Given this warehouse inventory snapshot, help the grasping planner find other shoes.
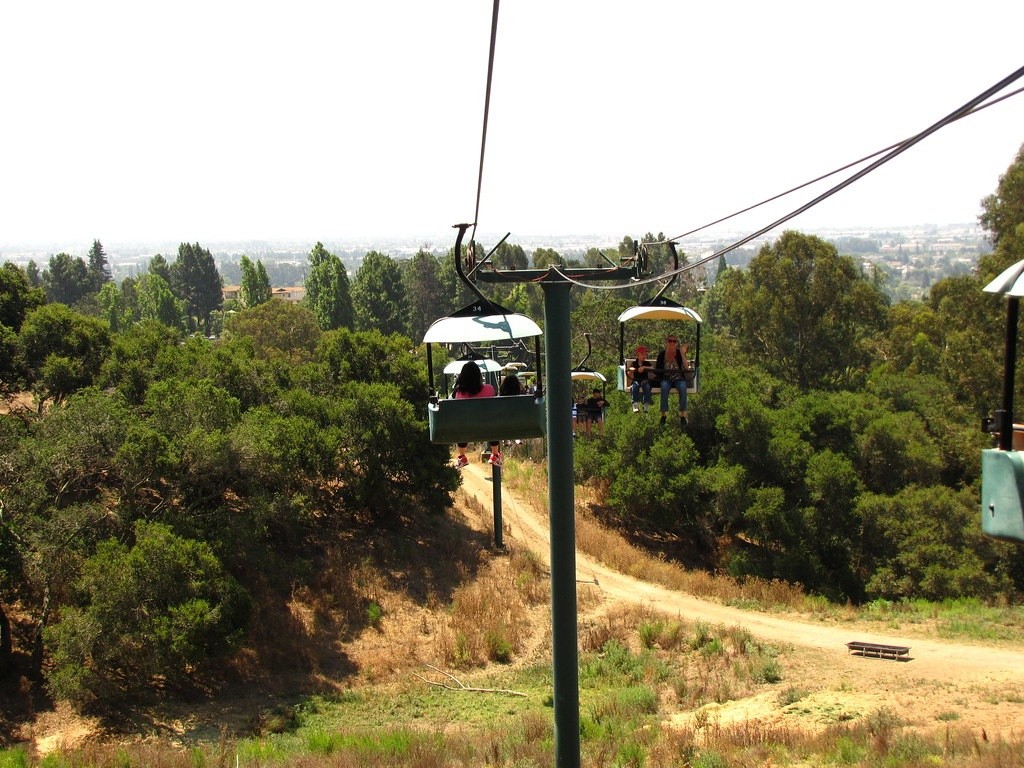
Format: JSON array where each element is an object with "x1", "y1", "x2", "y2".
[
  {"x1": 660, "y1": 415, "x2": 666, "y2": 425},
  {"x1": 633, "y1": 403, "x2": 639, "y2": 413},
  {"x1": 643, "y1": 403, "x2": 649, "y2": 412},
  {"x1": 680, "y1": 415, "x2": 688, "y2": 425}
]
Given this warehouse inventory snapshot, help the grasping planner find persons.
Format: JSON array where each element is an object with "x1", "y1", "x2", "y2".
[
  {"x1": 450, "y1": 362, "x2": 503, "y2": 468},
  {"x1": 629, "y1": 346, "x2": 654, "y2": 412},
  {"x1": 497, "y1": 375, "x2": 527, "y2": 446},
  {"x1": 654, "y1": 335, "x2": 690, "y2": 424},
  {"x1": 571, "y1": 389, "x2": 610, "y2": 438}
]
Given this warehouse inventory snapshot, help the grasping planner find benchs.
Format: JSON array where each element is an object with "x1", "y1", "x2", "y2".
[{"x1": 846, "y1": 641, "x2": 909, "y2": 660}]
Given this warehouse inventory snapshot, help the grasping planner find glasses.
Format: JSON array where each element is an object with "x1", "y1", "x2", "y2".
[{"x1": 667, "y1": 339, "x2": 677, "y2": 343}]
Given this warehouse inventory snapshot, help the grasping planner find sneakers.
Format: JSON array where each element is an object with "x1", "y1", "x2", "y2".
[
  {"x1": 453, "y1": 456, "x2": 469, "y2": 468},
  {"x1": 488, "y1": 453, "x2": 502, "y2": 466}
]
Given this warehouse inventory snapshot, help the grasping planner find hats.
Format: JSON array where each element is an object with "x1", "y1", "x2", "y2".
[
  {"x1": 636, "y1": 347, "x2": 649, "y2": 353},
  {"x1": 592, "y1": 388, "x2": 602, "y2": 393}
]
